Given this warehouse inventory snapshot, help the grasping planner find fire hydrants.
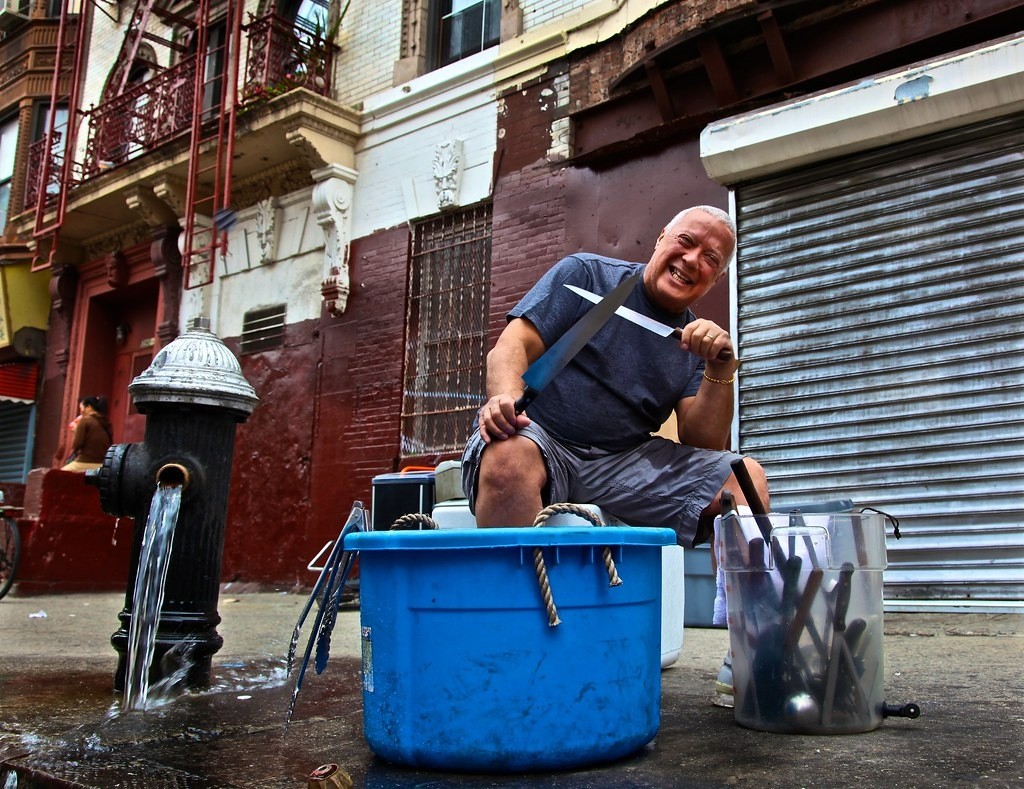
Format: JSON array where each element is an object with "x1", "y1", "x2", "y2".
[{"x1": 84, "y1": 320, "x2": 260, "y2": 696}]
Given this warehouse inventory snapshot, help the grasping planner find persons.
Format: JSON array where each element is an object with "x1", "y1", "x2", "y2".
[
  {"x1": 55, "y1": 395, "x2": 114, "y2": 473},
  {"x1": 463, "y1": 201, "x2": 818, "y2": 722}
]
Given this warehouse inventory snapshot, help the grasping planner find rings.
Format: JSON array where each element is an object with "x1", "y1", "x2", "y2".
[{"x1": 705, "y1": 334, "x2": 714, "y2": 340}]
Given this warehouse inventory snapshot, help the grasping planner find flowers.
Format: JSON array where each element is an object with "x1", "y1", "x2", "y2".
[{"x1": 235, "y1": 74, "x2": 300, "y2": 117}]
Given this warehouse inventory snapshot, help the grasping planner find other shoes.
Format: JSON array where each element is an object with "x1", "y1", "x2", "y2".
[{"x1": 710, "y1": 649, "x2": 734, "y2": 708}]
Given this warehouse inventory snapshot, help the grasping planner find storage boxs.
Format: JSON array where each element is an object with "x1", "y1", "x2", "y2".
[
  {"x1": 371, "y1": 474, "x2": 436, "y2": 530},
  {"x1": 683, "y1": 543, "x2": 729, "y2": 629},
  {"x1": 434, "y1": 460, "x2": 467, "y2": 502},
  {"x1": 433, "y1": 503, "x2": 684, "y2": 669}
]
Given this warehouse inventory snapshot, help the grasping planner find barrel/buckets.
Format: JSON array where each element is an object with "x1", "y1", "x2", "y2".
[{"x1": 718, "y1": 513, "x2": 888, "y2": 736}]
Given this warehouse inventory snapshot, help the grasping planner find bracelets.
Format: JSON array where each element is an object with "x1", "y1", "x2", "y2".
[{"x1": 702, "y1": 368, "x2": 736, "y2": 384}]
